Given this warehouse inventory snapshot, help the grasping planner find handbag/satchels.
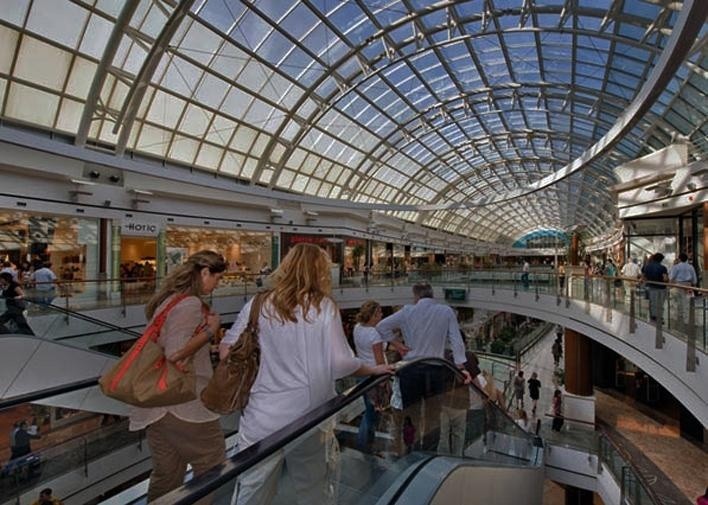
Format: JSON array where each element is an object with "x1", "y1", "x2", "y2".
[
  {"x1": 201, "y1": 332, "x2": 259, "y2": 415},
  {"x1": 375, "y1": 380, "x2": 393, "y2": 412},
  {"x1": 98, "y1": 326, "x2": 196, "y2": 407}
]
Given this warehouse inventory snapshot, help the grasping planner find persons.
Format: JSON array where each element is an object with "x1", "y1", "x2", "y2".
[
  {"x1": 1, "y1": 255, "x2": 157, "y2": 334},
  {"x1": 590, "y1": 251, "x2": 700, "y2": 325},
  {"x1": 224, "y1": 244, "x2": 395, "y2": 505},
  {"x1": 353, "y1": 280, "x2": 564, "y2": 458},
  {"x1": 7, "y1": 414, "x2": 122, "y2": 505},
  {"x1": 145, "y1": 250, "x2": 226, "y2": 505},
  {"x1": 256, "y1": 261, "x2": 270, "y2": 280},
  {"x1": 395, "y1": 257, "x2": 592, "y2": 299}
]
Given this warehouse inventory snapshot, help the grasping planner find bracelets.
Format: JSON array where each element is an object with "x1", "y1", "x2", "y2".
[{"x1": 206, "y1": 329, "x2": 215, "y2": 339}]
